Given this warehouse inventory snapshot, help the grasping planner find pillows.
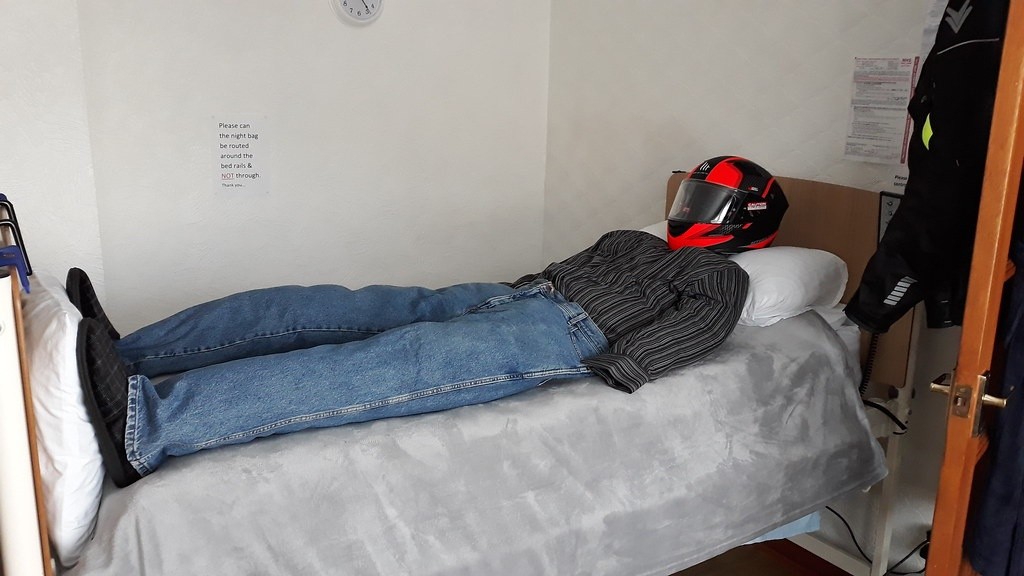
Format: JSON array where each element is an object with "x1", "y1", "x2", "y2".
[
  {"x1": 639, "y1": 219, "x2": 848, "y2": 327},
  {"x1": 21, "y1": 269, "x2": 107, "y2": 569}
]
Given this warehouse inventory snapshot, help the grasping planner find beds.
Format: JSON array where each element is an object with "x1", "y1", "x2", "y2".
[{"x1": 0, "y1": 171, "x2": 926, "y2": 576}]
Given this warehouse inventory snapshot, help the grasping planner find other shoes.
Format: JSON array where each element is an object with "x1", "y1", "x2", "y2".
[
  {"x1": 75, "y1": 320, "x2": 141, "y2": 487},
  {"x1": 66, "y1": 266, "x2": 119, "y2": 346}
]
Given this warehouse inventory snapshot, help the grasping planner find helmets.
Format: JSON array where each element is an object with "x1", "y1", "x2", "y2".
[{"x1": 666, "y1": 152, "x2": 790, "y2": 251}]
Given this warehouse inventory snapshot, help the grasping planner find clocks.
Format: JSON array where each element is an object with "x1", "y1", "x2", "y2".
[{"x1": 332, "y1": 0, "x2": 385, "y2": 27}]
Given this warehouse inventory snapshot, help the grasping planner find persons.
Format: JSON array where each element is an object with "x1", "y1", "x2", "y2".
[{"x1": 66, "y1": 155, "x2": 789, "y2": 487}]
